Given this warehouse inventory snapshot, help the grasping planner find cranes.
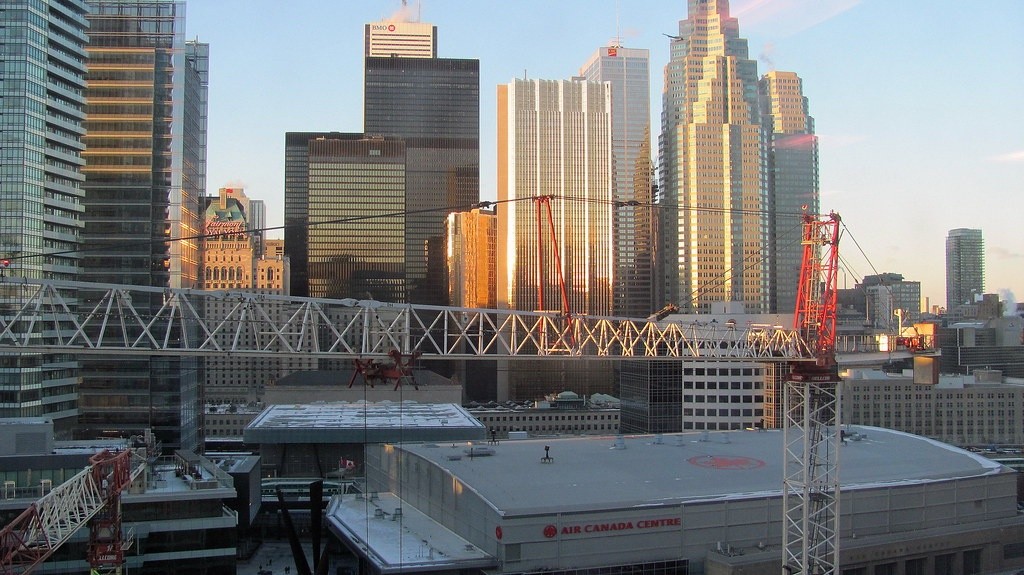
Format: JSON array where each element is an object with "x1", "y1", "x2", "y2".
[{"x1": 0, "y1": 192, "x2": 943, "y2": 574}]
